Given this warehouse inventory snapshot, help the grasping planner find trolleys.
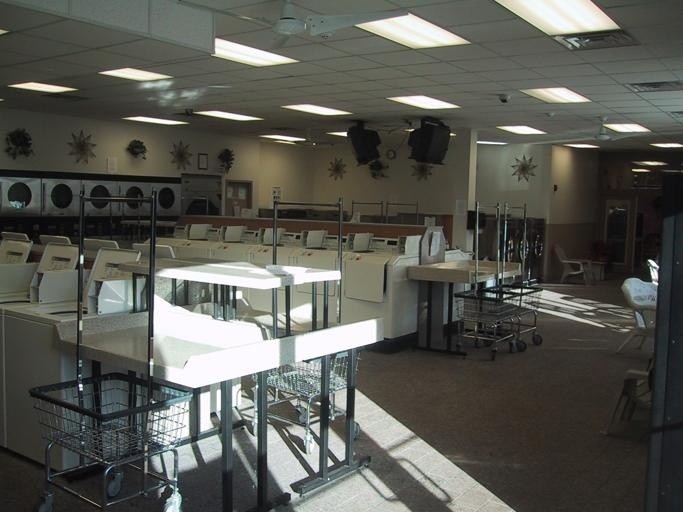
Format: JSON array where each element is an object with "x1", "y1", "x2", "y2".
[
  {"x1": 451, "y1": 284, "x2": 545, "y2": 363},
  {"x1": 28, "y1": 370, "x2": 193, "y2": 510},
  {"x1": 247, "y1": 347, "x2": 365, "y2": 457}
]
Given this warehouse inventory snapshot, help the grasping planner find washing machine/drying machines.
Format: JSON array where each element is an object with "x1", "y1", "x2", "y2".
[
  {"x1": 477, "y1": 215, "x2": 545, "y2": 284},
  {"x1": 0, "y1": 167, "x2": 475, "y2": 481}
]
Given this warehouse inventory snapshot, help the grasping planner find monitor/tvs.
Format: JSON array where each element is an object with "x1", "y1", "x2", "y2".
[
  {"x1": 343, "y1": 126, "x2": 382, "y2": 167},
  {"x1": 408, "y1": 125, "x2": 450, "y2": 165}
]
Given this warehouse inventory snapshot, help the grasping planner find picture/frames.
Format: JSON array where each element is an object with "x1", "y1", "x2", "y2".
[{"x1": 197, "y1": 153, "x2": 208, "y2": 170}]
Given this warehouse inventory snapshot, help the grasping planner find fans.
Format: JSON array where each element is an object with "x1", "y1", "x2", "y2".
[{"x1": 177, "y1": 0, "x2": 412, "y2": 53}]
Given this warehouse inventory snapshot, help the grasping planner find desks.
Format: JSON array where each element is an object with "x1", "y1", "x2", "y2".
[{"x1": 405, "y1": 258, "x2": 522, "y2": 357}]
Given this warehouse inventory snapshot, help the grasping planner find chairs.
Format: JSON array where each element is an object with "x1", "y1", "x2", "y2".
[
  {"x1": 551, "y1": 244, "x2": 593, "y2": 286},
  {"x1": 619, "y1": 276, "x2": 659, "y2": 351},
  {"x1": 646, "y1": 258, "x2": 660, "y2": 284}
]
